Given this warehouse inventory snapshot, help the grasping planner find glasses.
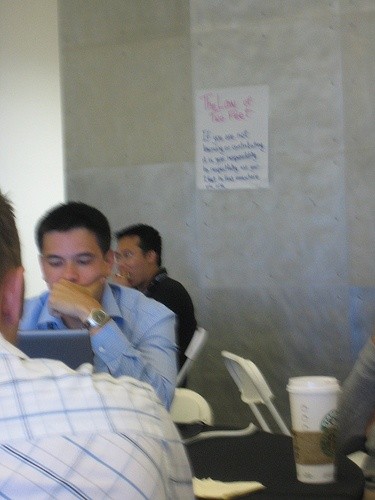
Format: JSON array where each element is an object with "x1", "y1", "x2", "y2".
[{"x1": 113, "y1": 247, "x2": 149, "y2": 265}]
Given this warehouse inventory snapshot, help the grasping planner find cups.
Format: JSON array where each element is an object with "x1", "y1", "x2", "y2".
[{"x1": 286, "y1": 374, "x2": 339, "y2": 485}]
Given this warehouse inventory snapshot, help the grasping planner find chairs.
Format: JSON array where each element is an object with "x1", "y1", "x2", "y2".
[{"x1": 168, "y1": 327, "x2": 291, "y2": 436}]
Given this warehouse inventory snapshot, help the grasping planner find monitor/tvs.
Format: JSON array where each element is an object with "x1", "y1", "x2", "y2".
[{"x1": 14, "y1": 329, "x2": 93, "y2": 370}]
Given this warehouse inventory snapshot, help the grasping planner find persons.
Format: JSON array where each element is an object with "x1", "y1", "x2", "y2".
[
  {"x1": 18, "y1": 201, "x2": 178, "y2": 411},
  {"x1": 322, "y1": 330, "x2": 375, "y2": 457},
  {"x1": 0, "y1": 194, "x2": 196, "y2": 500},
  {"x1": 113, "y1": 224, "x2": 197, "y2": 391}
]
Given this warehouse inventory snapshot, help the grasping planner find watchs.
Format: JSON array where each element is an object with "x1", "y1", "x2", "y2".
[{"x1": 84, "y1": 309, "x2": 110, "y2": 329}]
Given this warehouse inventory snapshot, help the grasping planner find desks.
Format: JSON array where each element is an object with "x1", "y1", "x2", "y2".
[{"x1": 177, "y1": 423, "x2": 365, "y2": 500}]
